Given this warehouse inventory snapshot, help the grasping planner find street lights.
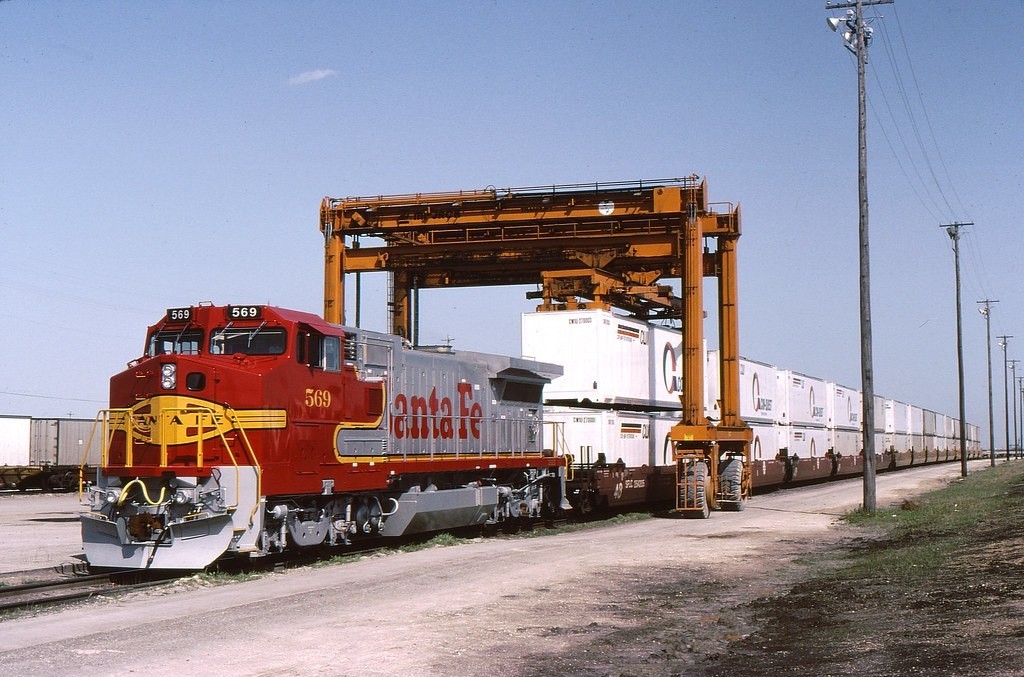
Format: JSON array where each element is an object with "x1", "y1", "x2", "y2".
[
  {"x1": 978, "y1": 309, "x2": 995, "y2": 468},
  {"x1": 998, "y1": 343, "x2": 1010, "y2": 462},
  {"x1": 1009, "y1": 364, "x2": 1021, "y2": 461},
  {"x1": 826, "y1": 14, "x2": 875, "y2": 518}
]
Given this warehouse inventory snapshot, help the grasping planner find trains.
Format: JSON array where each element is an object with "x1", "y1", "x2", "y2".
[{"x1": 56, "y1": 301, "x2": 980, "y2": 586}]
